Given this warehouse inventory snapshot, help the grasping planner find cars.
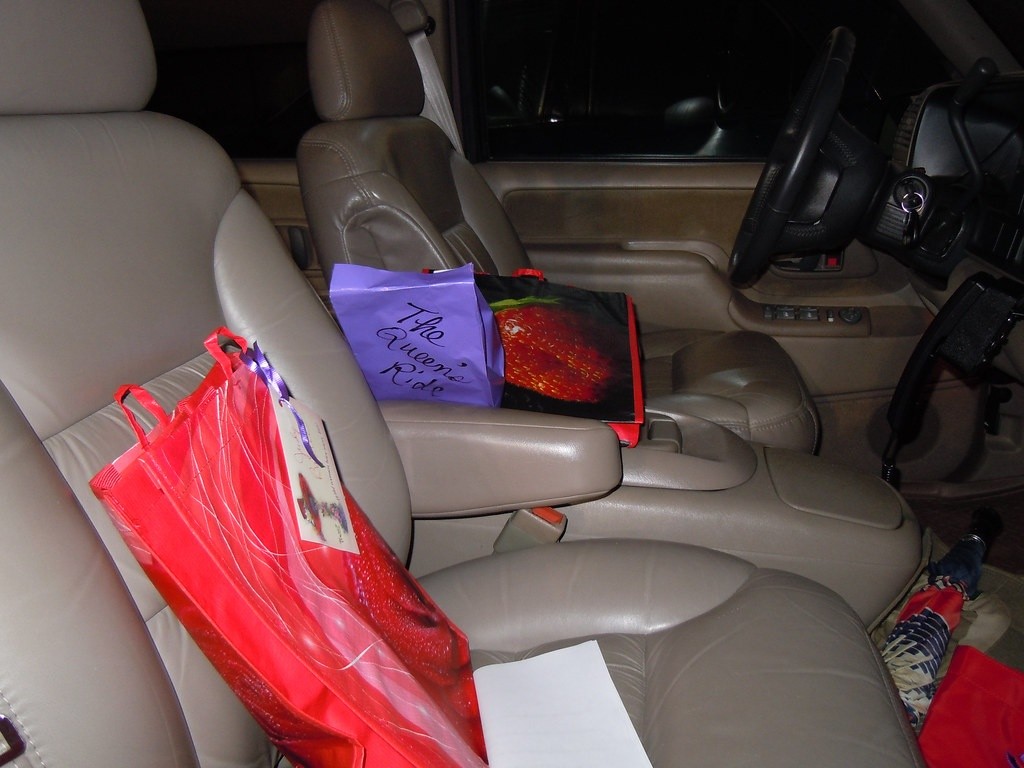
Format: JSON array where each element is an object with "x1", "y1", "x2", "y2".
[{"x1": 216, "y1": 0, "x2": 924, "y2": 155}]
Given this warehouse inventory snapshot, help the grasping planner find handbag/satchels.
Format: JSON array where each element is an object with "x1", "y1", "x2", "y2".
[
  {"x1": 421, "y1": 266, "x2": 644, "y2": 450},
  {"x1": 89, "y1": 324, "x2": 488, "y2": 768}
]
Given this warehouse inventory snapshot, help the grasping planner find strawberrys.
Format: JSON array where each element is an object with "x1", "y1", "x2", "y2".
[{"x1": 493, "y1": 304, "x2": 633, "y2": 404}]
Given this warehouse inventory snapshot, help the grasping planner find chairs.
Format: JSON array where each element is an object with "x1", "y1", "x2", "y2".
[{"x1": 0, "y1": 0, "x2": 927, "y2": 768}]
